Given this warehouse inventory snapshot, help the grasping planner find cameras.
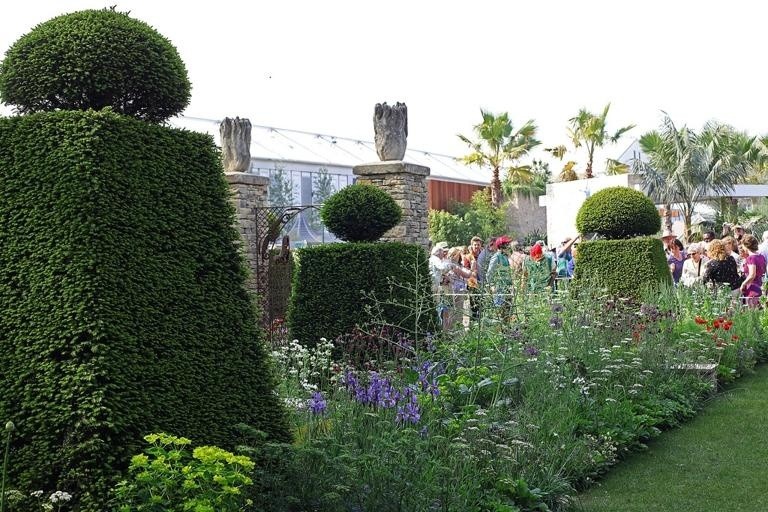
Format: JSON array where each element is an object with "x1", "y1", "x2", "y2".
[{"x1": 724, "y1": 223, "x2": 732, "y2": 228}]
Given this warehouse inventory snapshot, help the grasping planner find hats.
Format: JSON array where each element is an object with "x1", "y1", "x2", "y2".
[
  {"x1": 441, "y1": 242, "x2": 448, "y2": 252},
  {"x1": 496, "y1": 236, "x2": 513, "y2": 248},
  {"x1": 661, "y1": 230, "x2": 677, "y2": 238}
]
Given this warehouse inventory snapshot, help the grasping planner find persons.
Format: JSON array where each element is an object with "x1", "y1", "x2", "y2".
[
  {"x1": 662, "y1": 222, "x2": 768, "y2": 311},
  {"x1": 428, "y1": 234, "x2": 580, "y2": 334}
]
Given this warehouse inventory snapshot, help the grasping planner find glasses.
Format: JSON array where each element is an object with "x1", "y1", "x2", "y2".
[{"x1": 688, "y1": 253, "x2": 696, "y2": 256}]
